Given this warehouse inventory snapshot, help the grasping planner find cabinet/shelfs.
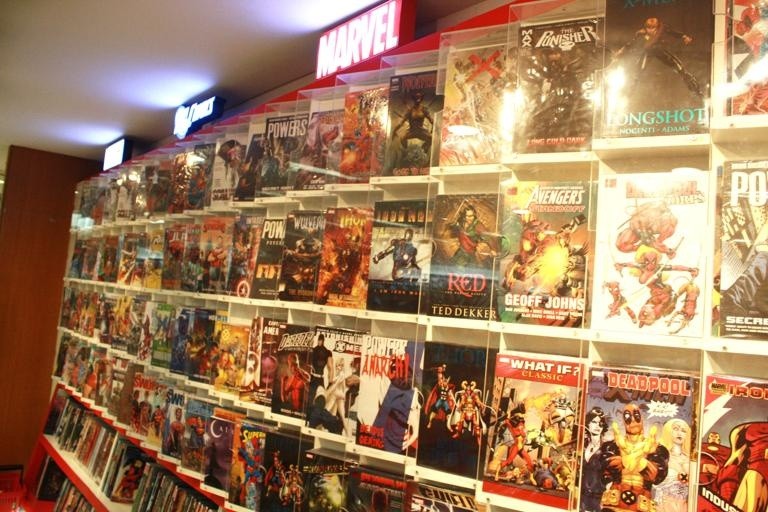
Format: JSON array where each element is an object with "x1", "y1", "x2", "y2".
[{"x1": 23, "y1": 0, "x2": 768, "y2": 511}]
